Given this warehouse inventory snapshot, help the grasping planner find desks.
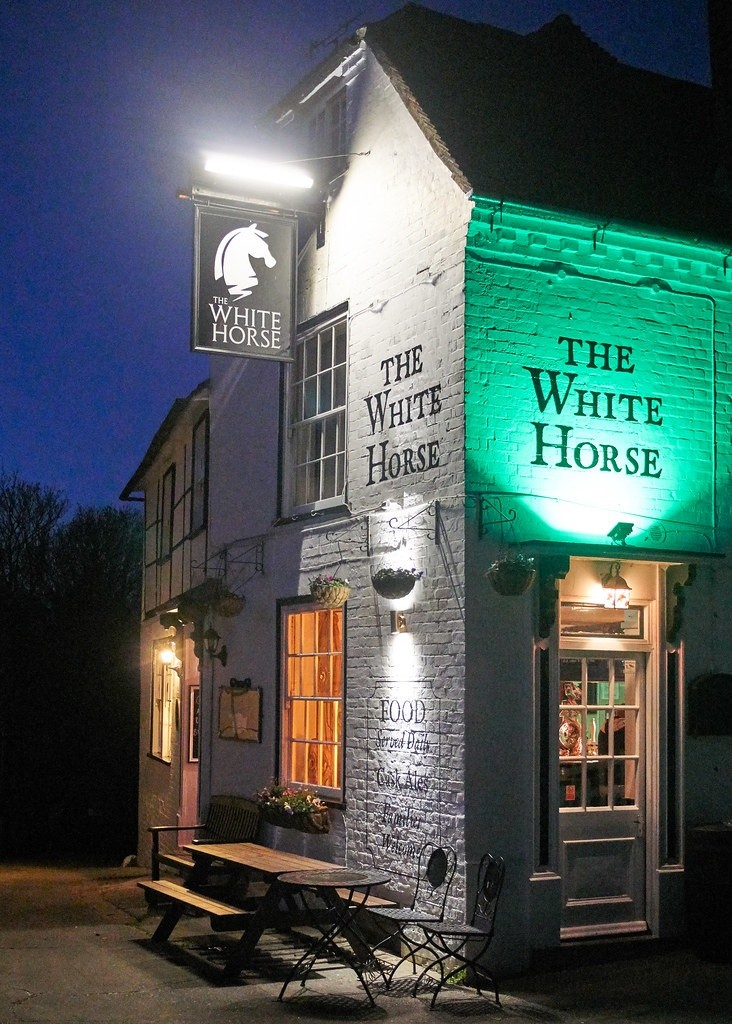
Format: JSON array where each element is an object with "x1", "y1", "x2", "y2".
[
  {"x1": 149, "y1": 842, "x2": 348, "y2": 982},
  {"x1": 276, "y1": 868, "x2": 391, "y2": 1008}
]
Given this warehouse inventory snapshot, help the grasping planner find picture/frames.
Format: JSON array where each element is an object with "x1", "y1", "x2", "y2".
[{"x1": 188, "y1": 684, "x2": 200, "y2": 763}]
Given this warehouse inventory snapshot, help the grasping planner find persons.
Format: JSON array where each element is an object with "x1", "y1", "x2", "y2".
[{"x1": 598, "y1": 703, "x2": 626, "y2": 806}]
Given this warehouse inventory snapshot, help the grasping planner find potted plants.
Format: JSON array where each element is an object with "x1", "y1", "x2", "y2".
[
  {"x1": 486, "y1": 553, "x2": 537, "y2": 597},
  {"x1": 177, "y1": 596, "x2": 208, "y2": 623},
  {"x1": 210, "y1": 586, "x2": 247, "y2": 617}
]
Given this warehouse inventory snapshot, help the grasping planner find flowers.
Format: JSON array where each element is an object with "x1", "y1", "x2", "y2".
[
  {"x1": 306, "y1": 573, "x2": 351, "y2": 588},
  {"x1": 254, "y1": 774, "x2": 328, "y2": 814},
  {"x1": 367, "y1": 567, "x2": 424, "y2": 580}
]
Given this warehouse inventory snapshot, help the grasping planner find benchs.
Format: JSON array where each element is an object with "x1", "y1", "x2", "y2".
[
  {"x1": 138, "y1": 879, "x2": 257, "y2": 982},
  {"x1": 310, "y1": 887, "x2": 398, "y2": 964}
]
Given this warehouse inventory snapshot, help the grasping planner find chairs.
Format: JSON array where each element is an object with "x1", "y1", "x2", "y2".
[
  {"x1": 412, "y1": 853, "x2": 506, "y2": 1010},
  {"x1": 144, "y1": 794, "x2": 261, "y2": 917},
  {"x1": 360, "y1": 841, "x2": 458, "y2": 993}
]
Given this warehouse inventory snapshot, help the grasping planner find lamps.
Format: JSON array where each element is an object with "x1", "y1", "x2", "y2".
[
  {"x1": 600, "y1": 561, "x2": 634, "y2": 612},
  {"x1": 203, "y1": 621, "x2": 228, "y2": 667},
  {"x1": 200, "y1": 150, "x2": 371, "y2": 190},
  {"x1": 389, "y1": 610, "x2": 406, "y2": 635},
  {"x1": 608, "y1": 520, "x2": 634, "y2": 547}
]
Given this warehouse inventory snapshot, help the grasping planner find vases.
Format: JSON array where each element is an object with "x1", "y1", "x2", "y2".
[
  {"x1": 372, "y1": 578, "x2": 416, "y2": 599},
  {"x1": 286, "y1": 812, "x2": 331, "y2": 835},
  {"x1": 258, "y1": 805, "x2": 292, "y2": 830},
  {"x1": 310, "y1": 588, "x2": 351, "y2": 608}
]
